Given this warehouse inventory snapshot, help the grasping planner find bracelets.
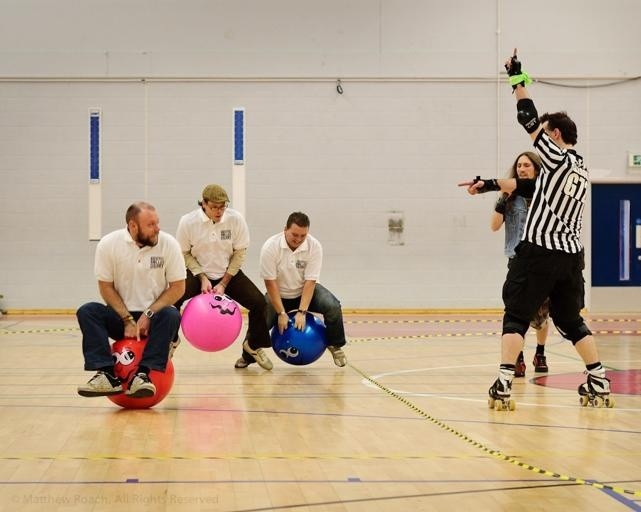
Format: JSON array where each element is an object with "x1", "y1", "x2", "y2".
[
  {"x1": 220, "y1": 281, "x2": 227, "y2": 288},
  {"x1": 121, "y1": 315, "x2": 135, "y2": 320},
  {"x1": 278, "y1": 311, "x2": 286, "y2": 315}
]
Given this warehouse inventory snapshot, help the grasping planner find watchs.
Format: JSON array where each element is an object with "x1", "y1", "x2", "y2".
[
  {"x1": 299, "y1": 309, "x2": 307, "y2": 315},
  {"x1": 144, "y1": 308, "x2": 155, "y2": 319}
]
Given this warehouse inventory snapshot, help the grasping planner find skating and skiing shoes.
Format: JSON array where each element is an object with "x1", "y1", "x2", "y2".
[
  {"x1": 577, "y1": 366, "x2": 615, "y2": 408},
  {"x1": 488, "y1": 369, "x2": 515, "y2": 411}
]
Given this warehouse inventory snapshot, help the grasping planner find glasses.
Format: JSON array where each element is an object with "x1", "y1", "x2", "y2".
[{"x1": 208, "y1": 203, "x2": 228, "y2": 211}]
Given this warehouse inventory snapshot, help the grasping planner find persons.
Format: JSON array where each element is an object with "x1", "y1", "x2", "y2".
[
  {"x1": 457, "y1": 48, "x2": 616, "y2": 399},
  {"x1": 492, "y1": 151, "x2": 549, "y2": 378},
  {"x1": 76, "y1": 201, "x2": 188, "y2": 398},
  {"x1": 169, "y1": 185, "x2": 275, "y2": 370},
  {"x1": 235, "y1": 212, "x2": 349, "y2": 369}
]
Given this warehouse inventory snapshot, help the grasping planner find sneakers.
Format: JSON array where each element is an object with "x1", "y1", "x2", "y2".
[
  {"x1": 532, "y1": 354, "x2": 548, "y2": 372},
  {"x1": 168, "y1": 337, "x2": 180, "y2": 360},
  {"x1": 235, "y1": 357, "x2": 248, "y2": 368},
  {"x1": 328, "y1": 346, "x2": 346, "y2": 366},
  {"x1": 243, "y1": 340, "x2": 273, "y2": 370},
  {"x1": 78, "y1": 370, "x2": 123, "y2": 397},
  {"x1": 125, "y1": 373, "x2": 156, "y2": 397},
  {"x1": 514, "y1": 360, "x2": 526, "y2": 377}
]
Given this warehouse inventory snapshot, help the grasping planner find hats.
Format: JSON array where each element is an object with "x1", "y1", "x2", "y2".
[{"x1": 202, "y1": 184, "x2": 230, "y2": 203}]
